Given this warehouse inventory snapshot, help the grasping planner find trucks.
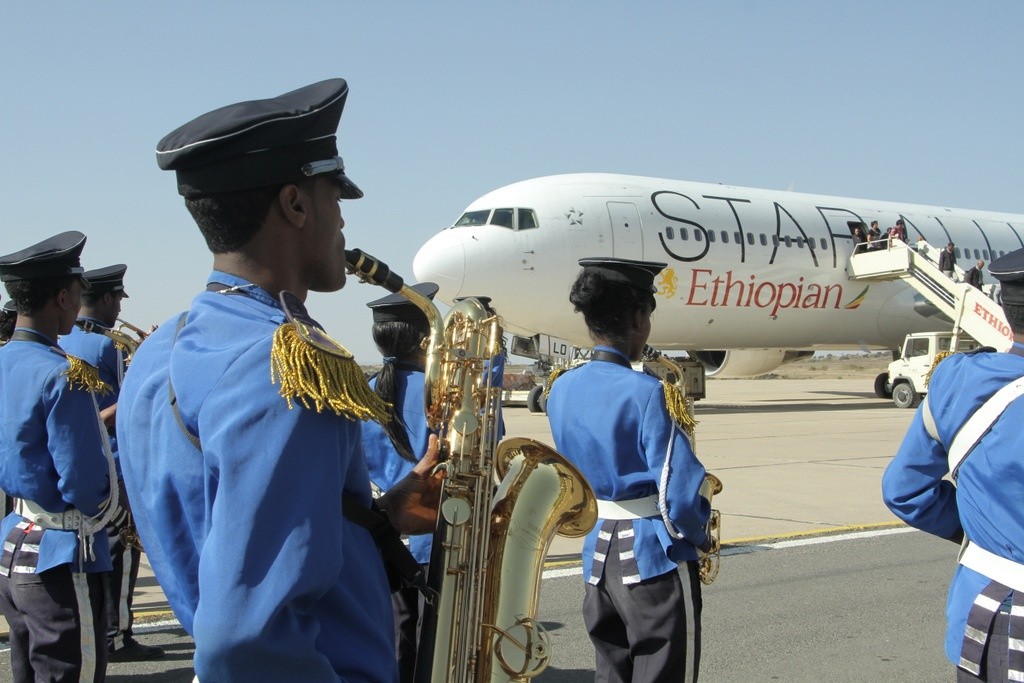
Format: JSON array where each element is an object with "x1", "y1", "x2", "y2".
[{"x1": 875, "y1": 330, "x2": 980, "y2": 408}]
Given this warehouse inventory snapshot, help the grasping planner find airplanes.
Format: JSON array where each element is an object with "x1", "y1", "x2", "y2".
[{"x1": 412, "y1": 170, "x2": 1024, "y2": 414}]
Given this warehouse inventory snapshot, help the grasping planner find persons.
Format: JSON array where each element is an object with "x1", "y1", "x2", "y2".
[
  {"x1": 548, "y1": 259, "x2": 721, "y2": 683},
  {"x1": 444, "y1": 296, "x2": 507, "y2": 495},
  {"x1": 0, "y1": 226, "x2": 162, "y2": 683},
  {"x1": 854, "y1": 219, "x2": 1003, "y2": 305},
  {"x1": 359, "y1": 282, "x2": 447, "y2": 683},
  {"x1": 119, "y1": 77, "x2": 448, "y2": 683},
  {"x1": 883, "y1": 249, "x2": 1024, "y2": 683}
]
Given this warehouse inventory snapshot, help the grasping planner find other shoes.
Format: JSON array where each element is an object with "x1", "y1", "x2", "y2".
[{"x1": 107, "y1": 641, "x2": 166, "y2": 661}]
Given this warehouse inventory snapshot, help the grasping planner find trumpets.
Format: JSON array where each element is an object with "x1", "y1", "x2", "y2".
[{"x1": 116, "y1": 318, "x2": 148, "y2": 344}]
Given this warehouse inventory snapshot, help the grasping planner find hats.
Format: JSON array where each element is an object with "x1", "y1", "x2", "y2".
[
  {"x1": 79, "y1": 263, "x2": 129, "y2": 299},
  {"x1": 3, "y1": 301, "x2": 18, "y2": 317},
  {"x1": 366, "y1": 282, "x2": 438, "y2": 322},
  {"x1": 578, "y1": 254, "x2": 671, "y2": 312},
  {"x1": 459, "y1": 294, "x2": 494, "y2": 314},
  {"x1": 0, "y1": 230, "x2": 93, "y2": 289},
  {"x1": 153, "y1": 78, "x2": 364, "y2": 199},
  {"x1": 988, "y1": 245, "x2": 1024, "y2": 308}
]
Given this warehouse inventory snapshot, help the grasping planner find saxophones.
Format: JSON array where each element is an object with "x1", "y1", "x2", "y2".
[
  {"x1": 642, "y1": 343, "x2": 723, "y2": 585},
  {"x1": 73, "y1": 318, "x2": 146, "y2": 553},
  {"x1": 344, "y1": 248, "x2": 598, "y2": 683}
]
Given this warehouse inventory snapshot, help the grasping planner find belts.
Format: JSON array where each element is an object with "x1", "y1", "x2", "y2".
[
  {"x1": 13, "y1": 497, "x2": 100, "y2": 531},
  {"x1": 960, "y1": 539, "x2": 1024, "y2": 593},
  {"x1": 597, "y1": 496, "x2": 667, "y2": 520}
]
